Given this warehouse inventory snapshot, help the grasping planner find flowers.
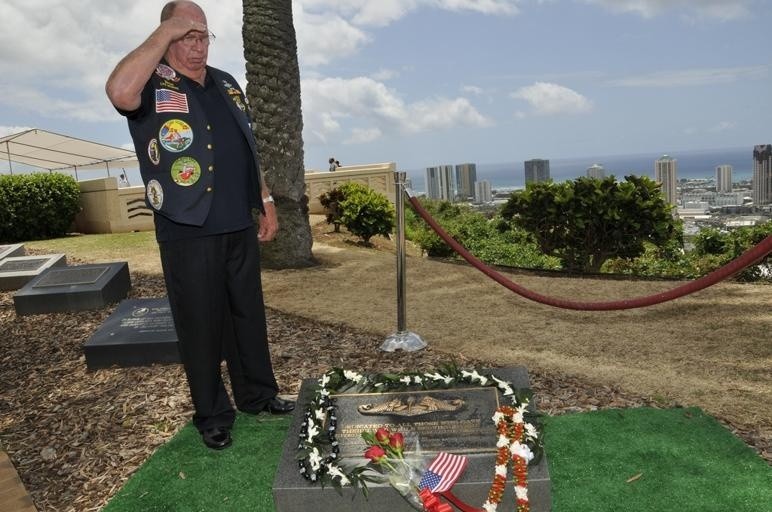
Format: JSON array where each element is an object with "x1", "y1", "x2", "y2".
[{"x1": 294, "y1": 367, "x2": 545, "y2": 511}]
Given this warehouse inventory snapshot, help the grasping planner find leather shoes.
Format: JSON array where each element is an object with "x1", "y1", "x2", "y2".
[
  {"x1": 261, "y1": 396, "x2": 296, "y2": 414},
  {"x1": 203, "y1": 427, "x2": 232, "y2": 450}
]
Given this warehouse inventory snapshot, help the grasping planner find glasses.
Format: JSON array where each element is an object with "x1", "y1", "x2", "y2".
[{"x1": 181, "y1": 30, "x2": 216, "y2": 46}]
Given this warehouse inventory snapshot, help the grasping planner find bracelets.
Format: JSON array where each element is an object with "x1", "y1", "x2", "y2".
[{"x1": 263, "y1": 196, "x2": 275, "y2": 204}]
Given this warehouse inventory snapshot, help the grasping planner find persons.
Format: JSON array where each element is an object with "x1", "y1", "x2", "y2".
[
  {"x1": 105, "y1": 0, "x2": 295, "y2": 449},
  {"x1": 329, "y1": 158, "x2": 338, "y2": 172},
  {"x1": 334, "y1": 160, "x2": 341, "y2": 168},
  {"x1": 118, "y1": 173, "x2": 129, "y2": 188}
]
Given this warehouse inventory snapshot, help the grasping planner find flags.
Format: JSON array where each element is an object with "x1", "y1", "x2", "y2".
[{"x1": 418, "y1": 450, "x2": 469, "y2": 493}]
